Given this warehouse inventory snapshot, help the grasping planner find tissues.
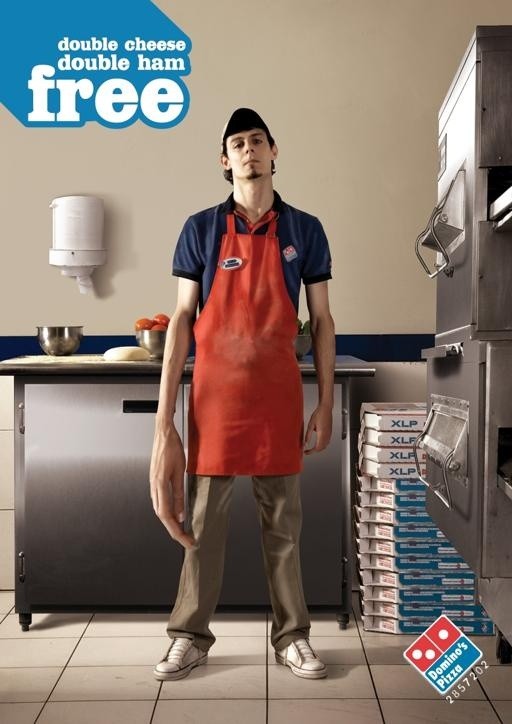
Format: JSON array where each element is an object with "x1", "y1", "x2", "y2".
[{"x1": 50, "y1": 196, "x2": 103, "y2": 298}]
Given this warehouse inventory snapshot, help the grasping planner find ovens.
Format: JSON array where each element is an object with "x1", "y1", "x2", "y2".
[{"x1": 414, "y1": 38, "x2": 486, "y2": 575}]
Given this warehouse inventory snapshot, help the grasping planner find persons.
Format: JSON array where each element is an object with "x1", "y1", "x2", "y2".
[{"x1": 148, "y1": 106, "x2": 337, "y2": 682}]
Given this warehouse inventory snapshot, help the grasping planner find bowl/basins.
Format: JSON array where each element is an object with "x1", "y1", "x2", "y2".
[
  {"x1": 135, "y1": 328, "x2": 167, "y2": 359},
  {"x1": 36, "y1": 322, "x2": 84, "y2": 355},
  {"x1": 295, "y1": 334, "x2": 313, "y2": 360}
]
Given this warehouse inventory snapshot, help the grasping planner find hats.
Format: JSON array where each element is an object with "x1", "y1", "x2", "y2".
[{"x1": 221, "y1": 107, "x2": 275, "y2": 152}]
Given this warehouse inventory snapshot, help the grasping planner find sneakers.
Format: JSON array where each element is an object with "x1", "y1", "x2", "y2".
[
  {"x1": 275, "y1": 638, "x2": 328, "y2": 679},
  {"x1": 153, "y1": 637, "x2": 208, "y2": 681}
]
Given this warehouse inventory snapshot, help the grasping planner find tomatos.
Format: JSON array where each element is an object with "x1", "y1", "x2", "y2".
[{"x1": 136, "y1": 314, "x2": 170, "y2": 331}]
